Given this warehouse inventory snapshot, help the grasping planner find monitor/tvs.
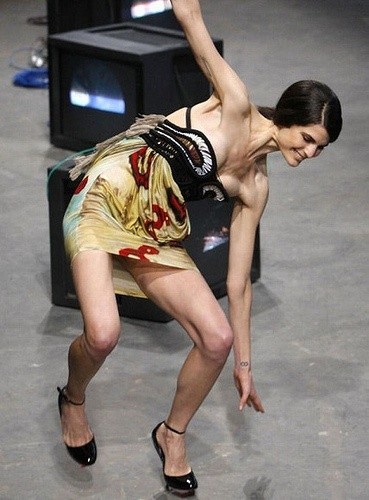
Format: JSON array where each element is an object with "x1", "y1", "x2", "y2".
[
  {"x1": 46, "y1": 0, "x2": 183, "y2": 35},
  {"x1": 46, "y1": 21, "x2": 224, "y2": 154},
  {"x1": 47, "y1": 160, "x2": 262, "y2": 324}
]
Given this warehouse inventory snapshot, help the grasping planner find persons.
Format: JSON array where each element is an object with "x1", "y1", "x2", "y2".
[{"x1": 55, "y1": 0, "x2": 343, "y2": 498}]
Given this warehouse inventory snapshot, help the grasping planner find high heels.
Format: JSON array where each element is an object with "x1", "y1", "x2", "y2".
[
  {"x1": 151, "y1": 420, "x2": 199, "y2": 491},
  {"x1": 56, "y1": 386, "x2": 98, "y2": 466}
]
